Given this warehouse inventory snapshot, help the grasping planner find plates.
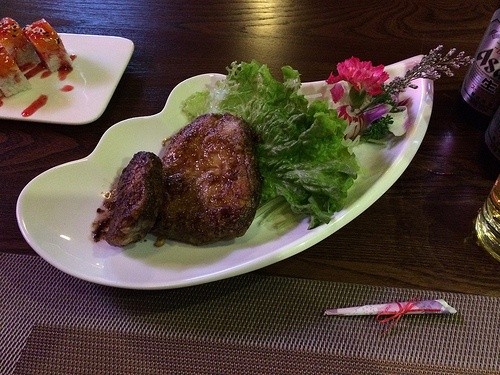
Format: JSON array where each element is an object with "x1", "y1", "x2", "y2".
[{"x1": 17, "y1": 54, "x2": 435, "y2": 289}]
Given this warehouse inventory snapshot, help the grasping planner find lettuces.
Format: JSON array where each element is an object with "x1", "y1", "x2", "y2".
[{"x1": 182, "y1": 59, "x2": 360, "y2": 231}]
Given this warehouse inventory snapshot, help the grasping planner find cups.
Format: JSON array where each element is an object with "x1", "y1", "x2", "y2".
[
  {"x1": 473, "y1": 174, "x2": 500, "y2": 260},
  {"x1": 0, "y1": 33, "x2": 135, "y2": 126}
]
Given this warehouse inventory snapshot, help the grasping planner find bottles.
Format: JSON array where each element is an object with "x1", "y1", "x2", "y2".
[{"x1": 455, "y1": 0, "x2": 500, "y2": 173}]
